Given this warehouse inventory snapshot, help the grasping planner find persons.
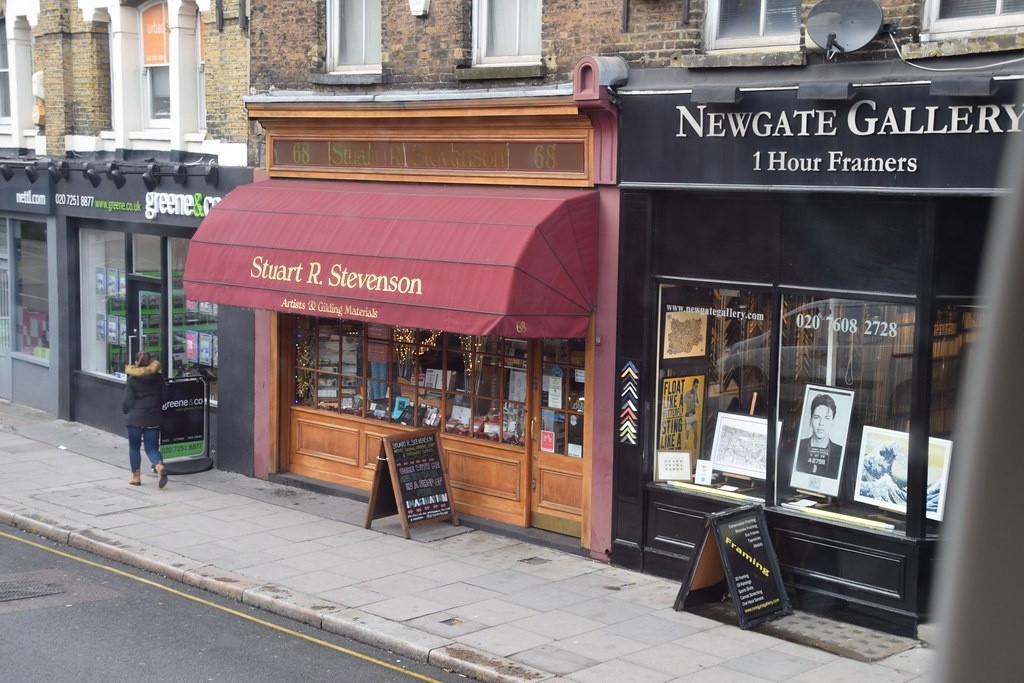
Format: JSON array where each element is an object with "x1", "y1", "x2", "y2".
[
  {"x1": 795, "y1": 394, "x2": 844, "y2": 480},
  {"x1": 121, "y1": 352, "x2": 168, "y2": 489},
  {"x1": 683, "y1": 379, "x2": 700, "y2": 459}
]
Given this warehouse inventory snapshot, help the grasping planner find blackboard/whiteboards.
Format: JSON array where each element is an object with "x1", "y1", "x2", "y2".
[
  {"x1": 382, "y1": 425, "x2": 456, "y2": 527},
  {"x1": 710, "y1": 502, "x2": 795, "y2": 630}
]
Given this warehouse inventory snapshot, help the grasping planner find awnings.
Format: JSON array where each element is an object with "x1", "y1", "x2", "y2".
[{"x1": 184, "y1": 177, "x2": 601, "y2": 337}]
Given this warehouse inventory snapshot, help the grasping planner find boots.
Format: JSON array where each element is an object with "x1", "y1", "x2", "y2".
[
  {"x1": 155, "y1": 462, "x2": 168, "y2": 488},
  {"x1": 129, "y1": 469, "x2": 141, "y2": 486}
]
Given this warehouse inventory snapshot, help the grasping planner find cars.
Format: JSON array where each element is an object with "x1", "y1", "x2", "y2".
[{"x1": 716, "y1": 300, "x2": 916, "y2": 417}]
